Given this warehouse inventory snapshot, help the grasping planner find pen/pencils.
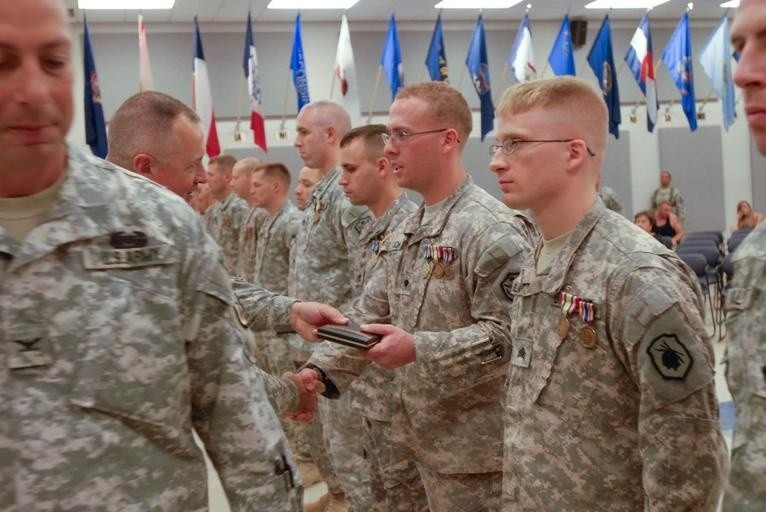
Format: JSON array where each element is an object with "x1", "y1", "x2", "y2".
[
  {"x1": 471, "y1": 337, "x2": 503, "y2": 365},
  {"x1": 275, "y1": 455, "x2": 296, "y2": 491}
]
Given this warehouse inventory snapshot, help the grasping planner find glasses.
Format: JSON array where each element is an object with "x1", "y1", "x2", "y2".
[
  {"x1": 381, "y1": 128, "x2": 461, "y2": 145},
  {"x1": 488, "y1": 136, "x2": 596, "y2": 157}
]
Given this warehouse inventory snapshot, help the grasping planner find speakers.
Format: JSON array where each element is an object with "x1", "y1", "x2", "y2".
[{"x1": 570, "y1": 17, "x2": 588, "y2": 46}]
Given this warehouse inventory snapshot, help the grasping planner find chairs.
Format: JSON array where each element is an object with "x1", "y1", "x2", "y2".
[{"x1": 654, "y1": 228, "x2": 756, "y2": 343}]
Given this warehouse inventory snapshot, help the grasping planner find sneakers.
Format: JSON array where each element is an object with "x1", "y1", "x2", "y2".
[
  {"x1": 299, "y1": 463, "x2": 322, "y2": 487},
  {"x1": 303, "y1": 492, "x2": 328, "y2": 511},
  {"x1": 322, "y1": 495, "x2": 348, "y2": 511}
]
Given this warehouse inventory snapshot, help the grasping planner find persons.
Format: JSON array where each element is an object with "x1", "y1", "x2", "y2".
[{"x1": 0, "y1": 1, "x2": 765, "y2": 512}]
